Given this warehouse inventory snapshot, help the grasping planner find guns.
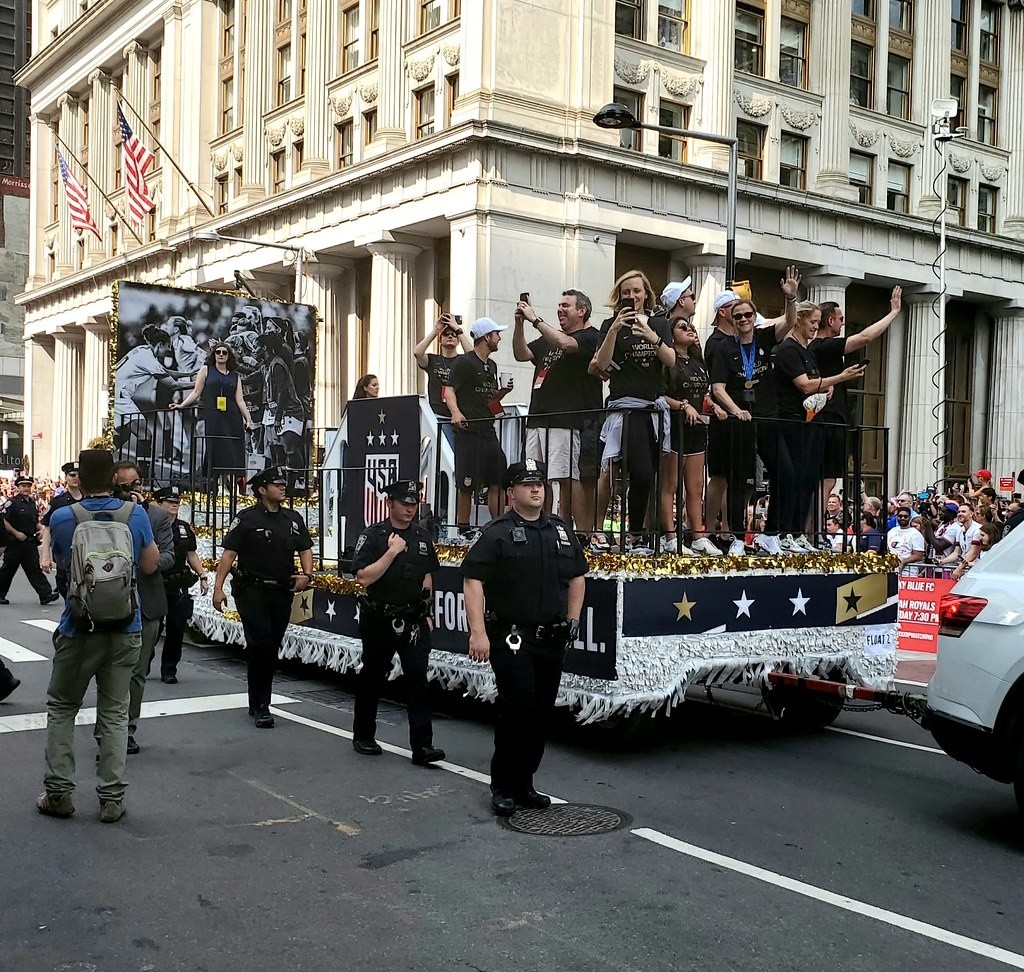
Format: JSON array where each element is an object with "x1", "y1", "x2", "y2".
[
  {"x1": 356, "y1": 594, "x2": 378, "y2": 638},
  {"x1": 230, "y1": 567, "x2": 243, "y2": 599}
]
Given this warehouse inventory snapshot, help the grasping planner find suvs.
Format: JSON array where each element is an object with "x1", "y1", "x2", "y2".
[{"x1": 925, "y1": 518, "x2": 1024, "y2": 823}]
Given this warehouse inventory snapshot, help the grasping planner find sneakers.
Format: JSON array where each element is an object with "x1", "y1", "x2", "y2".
[
  {"x1": 100, "y1": 799, "x2": 126, "y2": 822},
  {"x1": 582, "y1": 529, "x2": 822, "y2": 558},
  {"x1": 36, "y1": 792, "x2": 75, "y2": 816}
]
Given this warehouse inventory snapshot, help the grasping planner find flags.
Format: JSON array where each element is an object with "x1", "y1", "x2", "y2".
[
  {"x1": 55, "y1": 146, "x2": 103, "y2": 242},
  {"x1": 117, "y1": 100, "x2": 156, "y2": 227}
]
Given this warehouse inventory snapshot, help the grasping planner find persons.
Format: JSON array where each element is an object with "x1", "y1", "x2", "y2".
[
  {"x1": 353, "y1": 374, "x2": 380, "y2": 400},
  {"x1": 703, "y1": 265, "x2": 907, "y2": 557},
  {"x1": 588, "y1": 269, "x2": 724, "y2": 560},
  {"x1": 0, "y1": 476, "x2": 68, "y2": 574},
  {"x1": 350, "y1": 477, "x2": 447, "y2": 766},
  {"x1": 115, "y1": 304, "x2": 312, "y2": 498},
  {"x1": 415, "y1": 313, "x2": 515, "y2": 544},
  {"x1": 0, "y1": 659, "x2": 21, "y2": 701},
  {"x1": 36, "y1": 448, "x2": 159, "y2": 824},
  {"x1": 514, "y1": 286, "x2": 604, "y2": 551},
  {"x1": 460, "y1": 459, "x2": 591, "y2": 814},
  {"x1": 38, "y1": 460, "x2": 93, "y2": 610},
  {"x1": 170, "y1": 342, "x2": 254, "y2": 496},
  {"x1": 211, "y1": 464, "x2": 315, "y2": 728},
  {"x1": 0, "y1": 475, "x2": 60, "y2": 605},
  {"x1": 623, "y1": 468, "x2": 1024, "y2": 580},
  {"x1": 145, "y1": 485, "x2": 210, "y2": 687},
  {"x1": 94, "y1": 460, "x2": 176, "y2": 763}
]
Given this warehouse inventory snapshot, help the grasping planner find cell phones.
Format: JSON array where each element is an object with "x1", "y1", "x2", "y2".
[
  {"x1": 244, "y1": 424, "x2": 252, "y2": 435},
  {"x1": 442, "y1": 315, "x2": 462, "y2": 325},
  {"x1": 856, "y1": 358, "x2": 871, "y2": 373},
  {"x1": 519, "y1": 292, "x2": 530, "y2": 315},
  {"x1": 622, "y1": 298, "x2": 635, "y2": 327}
]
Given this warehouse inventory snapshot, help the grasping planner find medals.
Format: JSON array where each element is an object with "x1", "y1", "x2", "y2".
[{"x1": 745, "y1": 380, "x2": 753, "y2": 388}]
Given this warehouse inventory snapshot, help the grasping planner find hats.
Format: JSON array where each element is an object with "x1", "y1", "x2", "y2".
[
  {"x1": 710, "y1": 290, "x2": 739, "y2": 326},
  {"x1": 61, "y1": 461, "x2": 79, "y2": 474},
  {"x1": 384, "y1": 479, "x2": 425, "y2": 504},
  {"x1": 245, "y1": 466, "x2": 286, "y2": 487},
  {"x1": 971, "y1": 469, "x2": 992, "y2": 480},
  {"x1": 659, "y1": 275, "x2": 692, "y2": 311},
  {"x1": 470, "y1": 318, "x2": 508, "y2": 340},
  {"x1": 152, "y1": 487, "x2": 181, "y2": 503},
  {"x1": 15, "y1": 476, "x2": 34, "y2": 486},
  {"x1": 503, "y1": 460, "x2": 547, "y2": 486}
]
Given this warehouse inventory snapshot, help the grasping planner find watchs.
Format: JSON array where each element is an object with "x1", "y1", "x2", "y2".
[
  {"x1": 533, "y1": 316, "x2": 544, "y2": 329},
  {"x1": 305, "y1": 573, "x2": 314, "y2": 582}
]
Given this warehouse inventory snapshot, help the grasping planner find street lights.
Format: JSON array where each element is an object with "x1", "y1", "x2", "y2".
[
  {"x1": 195, "y1": 232, "x2": 304, "y2": 306},
  {"x1": 931, "y1": 98, "x2": 970, "y2": 498},
  {"x1": 593, "y1": 101, "x2": 737, "y2": 295}
]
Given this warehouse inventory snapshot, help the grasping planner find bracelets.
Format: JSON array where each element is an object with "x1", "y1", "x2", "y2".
[
  {"x1": 680, "y1": 399, "x2": 690, "y2": 411},
  {"x1": 455, "y1": 328, "x2": 463, "y2": 335},
  {"x1": 710, "y1": 403, "x2": 717, "y2": 414},
  {"x1": 653, "y1": 337, "x2": 663, "y2": 349},
  {"x1": 817, "y1": 376, "x2": 823, "y2": 393},
  {"x1": 200, "y1": 577, "x2": 207, "y2": 580}
]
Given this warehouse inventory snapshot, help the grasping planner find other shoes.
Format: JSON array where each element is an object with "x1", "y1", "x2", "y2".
[
  {"x1": 491, "y1": 789, "x2": 516, "y2": 816},
  {"x1": 126, "y1": 737, "x2": 139, "y2": 754},
  {"x1": 40, "y1": 593, "x2": 59, "y2": 606},
  {"x1": 0, "y1": 598, "x2": 10, "y2": 605},
  {"x1": 0, "y1": 678, "x2": 21, "y2": 701},
  {"x1": 517, "y1": 787, "x2": 552, "y2": 808},
  {"x1": 248, "y1": 704, "x2": 274, "y2": 727},
  {"x1": 353, "y1": 737, "x2": 383, "y2": 756},
  {"x1": 412, "y1": 745, "x2": 445, "y2": 765},
  {"x1": 160, "y1": 675, "x2": 178, "y2": 685}
]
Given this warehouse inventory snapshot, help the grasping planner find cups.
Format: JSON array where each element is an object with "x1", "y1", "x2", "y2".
[
  {"x1": 501, "y1": 372, "x2": 512, "y2": 388},
  {"x1": 632, "y1": 314, "x2": 649, "y2": 334}
]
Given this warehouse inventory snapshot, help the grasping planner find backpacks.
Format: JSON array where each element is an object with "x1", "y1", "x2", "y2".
[{"x1": 68, "y1": 502, "x2": 137, "y2": 628}]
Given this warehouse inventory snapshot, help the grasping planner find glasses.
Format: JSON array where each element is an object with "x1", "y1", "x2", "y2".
[
  {"x1": 214, "y1": 350, "x2": 229, "y2": 356},
  {"x1": 676, "y1": 323, "x2": 697, "y2": 332},
  {"x1": 442, "y1": 331, "x2": 457, "y2": 337},
  {"x1": 896, "y1": 500, "x2": 908, "y2": 502},
  {"x1": 683, "y1": 293, "x2": 696, "y2": 301},
  {"x1": 69, "y1": 473, "x2": 77, "y2": 477},
  {"x1": 733, "y1": 311, "x2": 754, "y2": 321},
  {"x1": 836, "y1": 315, "x2": 844, "y2": 323},
  {"x1": 898, "y1": 514, "x2": 909, "y2": 519}
]
